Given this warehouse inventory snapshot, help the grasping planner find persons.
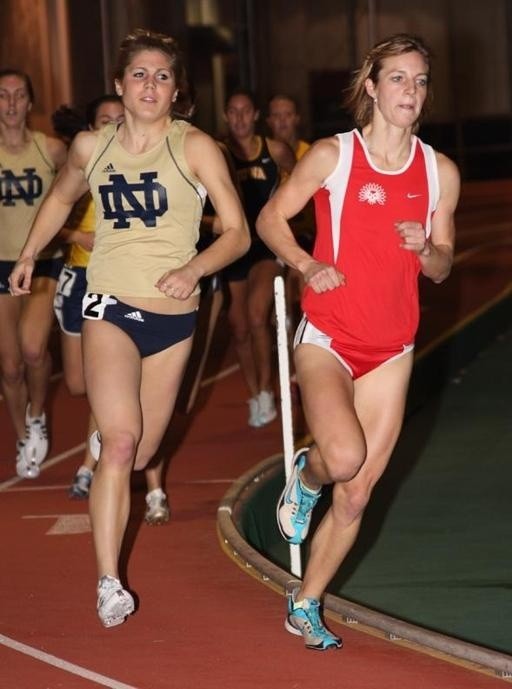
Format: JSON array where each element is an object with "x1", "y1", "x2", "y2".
[
  {"x1": 9, "y1": 29, "x2": 252, "y2": 631},
  {"x1": 0, "y1": 71, "x2": 65, "y2": 486},
  {"x1": 53, "y1": 94, "x2": 128, "y2": 499},
  {"x1": 253, "y1": 31, "x2": 462, "y2": 653},
  {"x1": 263, "y1": 90, "x2": 317, "y2": 188},
  {"x1": 143, "y1": 78, "x2": 233, "y2": 535},
  {"x1": 207, "y1": 79, "x2": 297, "y2": 427}
]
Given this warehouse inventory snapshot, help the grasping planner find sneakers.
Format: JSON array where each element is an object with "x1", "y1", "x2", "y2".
[
  {"x1": 16, "y1": 402, "x2": 51, "y2": 485},
  {"x1": 97, "y1": 575, "x2": 135, "y2": 628},
  {"x1": 248, "y1": 391, "x2": 278, "y2": 429},
  {"x1": 145, "y1": 488, "x2": 169, "y2": 527},
  {"x1": 284, "y1": 593, "x2": 343, "y2": 651},
  {"x1": 68, "y1": 467, "x2": 91, "y2": 498},
  {"x1": 277, "y1": 447, "x2": 322, "y2": 544}
]
